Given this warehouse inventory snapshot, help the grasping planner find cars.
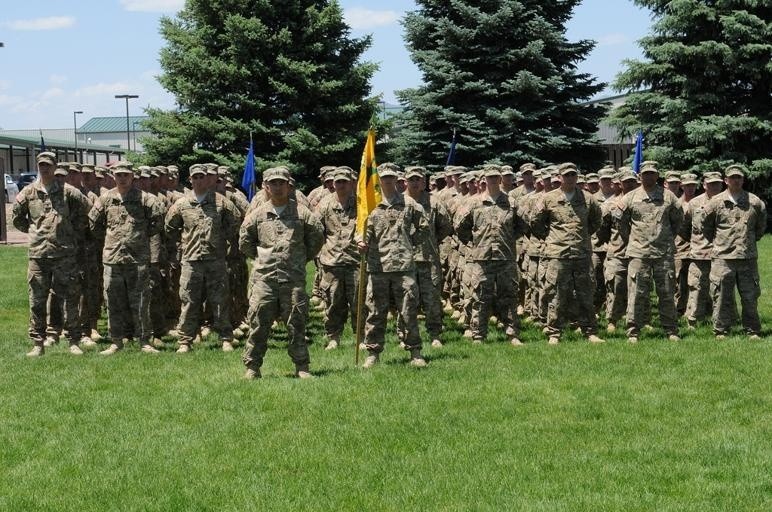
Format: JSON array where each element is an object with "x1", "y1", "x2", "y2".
[{"x1": 4, "y1": 172, "x2": 38, "y2": 203}]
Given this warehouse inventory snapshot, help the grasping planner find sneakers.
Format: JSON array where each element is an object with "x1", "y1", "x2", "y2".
[{"x1": 22, "y1": 305, "x2": 758, "y2": 357}]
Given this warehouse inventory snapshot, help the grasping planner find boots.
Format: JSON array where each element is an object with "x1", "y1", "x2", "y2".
[
  {"x1": 358, "y1": 352, "x2": 381, "y2": 369},
  {"x1": 289, "y1": 364, "x2": 319, "y2": 379},
  {"x1": 236, "y1": 367, "x2": 264, "y2": 380},
  {"x1": 406, "y1": 350, "x2": 428, "y2": 369}
]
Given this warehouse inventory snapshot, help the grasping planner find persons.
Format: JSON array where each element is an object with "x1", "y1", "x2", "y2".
[{"x1": 238, "y1": 167, "x2": 328, "y2": 379}]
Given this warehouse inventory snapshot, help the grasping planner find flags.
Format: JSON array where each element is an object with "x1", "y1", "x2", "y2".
[
  {"x1": 443, "y1": 140, "x2": 459, "y2": 166},
  {"x1": 239, "y1": 144, "x2": 259, "y2": 203},
  {"x1": 629, "y1": 135, "x2": 654, "y2": 169},
  {"x1": 353, "y1": 130, "x2": 388, "y2": 243}
]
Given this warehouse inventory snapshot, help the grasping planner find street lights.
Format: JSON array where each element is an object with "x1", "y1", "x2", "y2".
[
  {"x1": 73, "y1": 111, "x2": 85, "y2": 162},
  {"x1": 115, "y1": 94, "x2": 140, "y2": 151},
  {"x1": 132, "y1": 122, "x2": 142, "y2": 151}
]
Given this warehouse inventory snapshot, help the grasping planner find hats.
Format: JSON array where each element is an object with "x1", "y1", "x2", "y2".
[{"x1": 33, "y1": 152, "x2": 747, "y2": 184}]
